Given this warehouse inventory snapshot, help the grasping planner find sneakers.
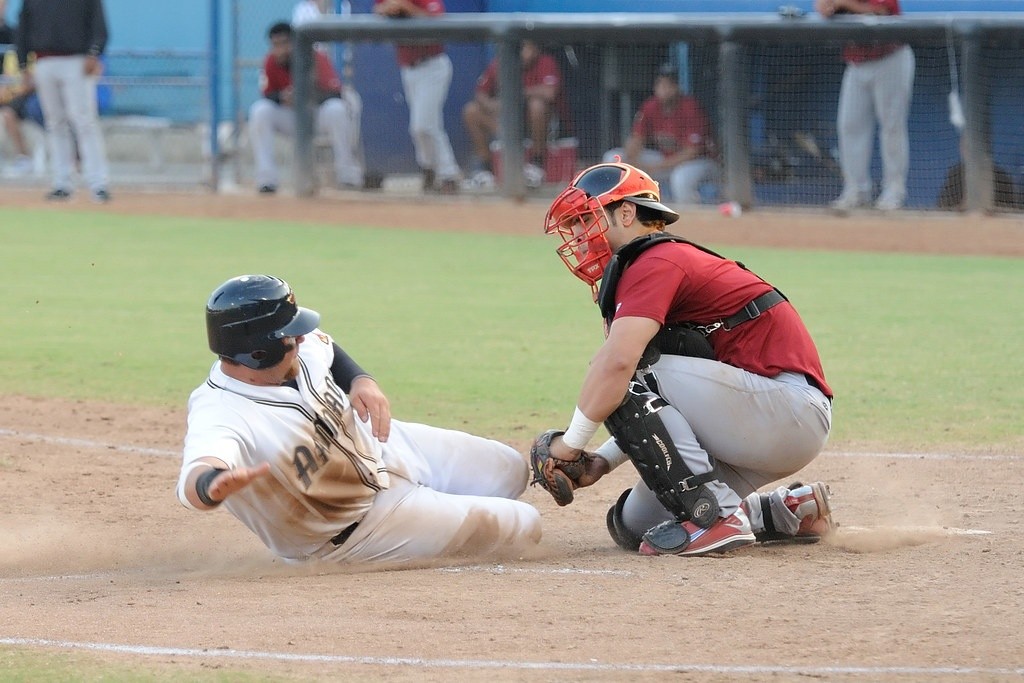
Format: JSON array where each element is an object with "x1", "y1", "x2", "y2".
[
  {"x1": 638, "y1": 502, "x2": 756, "y2": 556},
  {"x1": 785, "y1": 482, "x2": 839, "y2": 541}
]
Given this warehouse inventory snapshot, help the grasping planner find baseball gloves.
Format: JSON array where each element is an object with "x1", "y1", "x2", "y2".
[{"x1": 528, "y1": 428, "x2": 591, "y2": 509}]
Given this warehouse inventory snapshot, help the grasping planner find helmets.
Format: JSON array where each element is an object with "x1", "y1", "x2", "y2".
[
  {"x1": 569, "y1": 154, "x2": 680, "y2": 228},
  {"x1": 206, "y1": 274, "x2": 321, "y2": 370}
]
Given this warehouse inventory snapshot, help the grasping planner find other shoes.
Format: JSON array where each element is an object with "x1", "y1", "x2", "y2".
[
  {"x1": 830, "y1": 187, "x2": 909, "y2": 214},
  {"x1": 96, "y1": 188, "x2": 109, "y2": 202},
  {"x1": 46, "y1": 188, "x2": 71, "y2": 200}
]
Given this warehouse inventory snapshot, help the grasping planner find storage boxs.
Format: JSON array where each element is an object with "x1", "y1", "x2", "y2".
[{"x1": 485, "y1": 134, "x2": 581, "y2": 183}]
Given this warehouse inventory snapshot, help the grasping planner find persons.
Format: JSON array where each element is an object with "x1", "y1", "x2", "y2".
[
  {"x1": 247, "y1": 0, "x2": 916, "y2": 210},
  {"x1": 531, "y1": 155, "x2": 841, "y2": 556},
  {"x1": 176, "y1": 273, "x2": 542, "y2": 567},
  {"x1": 0, "y1": 0, "x2": 110, "y2": 202}
]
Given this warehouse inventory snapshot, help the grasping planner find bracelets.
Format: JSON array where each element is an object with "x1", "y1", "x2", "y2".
[
  {"x1": 563, "y1": 405, "x2": 606, "y2": 450},
  {"x1": 593, "y1": 434, "x2": 632, "y2": 473},
  {"x1": 196, "y1": 467, "x2": 227, "y2": 508}
]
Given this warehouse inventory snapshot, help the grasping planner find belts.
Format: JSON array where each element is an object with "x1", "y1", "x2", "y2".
[
  {"x1": 804, "y1": 373, "x2": 831, "y2": 403},
  {"x1": 326, "y1": 522, "x2": 358, "y2": 552}
]
